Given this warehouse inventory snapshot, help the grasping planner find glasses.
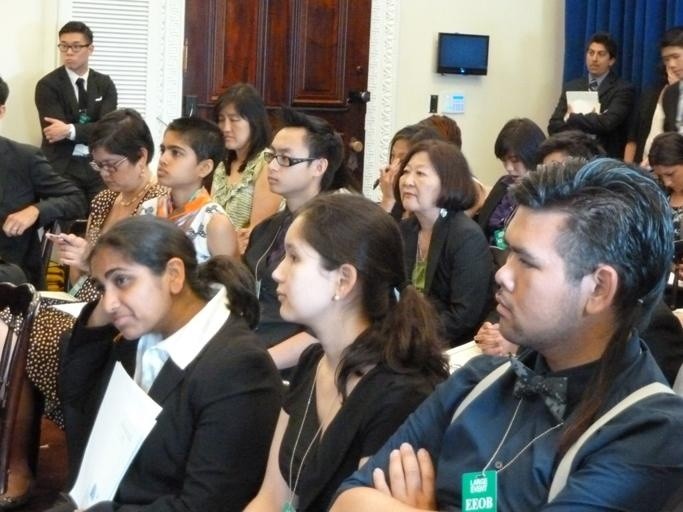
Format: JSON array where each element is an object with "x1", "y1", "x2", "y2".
[
  {"x1": 262, "y1": 151, "x2": 316, "y2": 168},
  {"x1": 88, "y1": 156, "x2": 131, "y2": 174},
  {"x1": 57, "y1": 42, "x2": 88, "y2": 53}
]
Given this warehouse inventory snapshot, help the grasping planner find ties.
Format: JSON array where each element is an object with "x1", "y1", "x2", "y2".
[
  {"x1": 76, "y1": 78, "x2": 88, "y2": 111},
  {"x1": 588, "y1": 78, "x2": 597, "y2": 91}
]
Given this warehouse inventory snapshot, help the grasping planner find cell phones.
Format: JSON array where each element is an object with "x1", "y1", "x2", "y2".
[{"x1": 50, "y1": 234, "x2": 73, "y2": 246}]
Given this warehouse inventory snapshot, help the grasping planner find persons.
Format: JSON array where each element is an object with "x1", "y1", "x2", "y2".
[
  {"x1": 623, "y1": 24, "x2": 683, "y2": 173},
  {"x1": 35, "y1": 18, "x2": 115, "y2": 196},
  {"x1": 547, "y1": 33, "x2": 639, "y2": 167},
  {"x1": 2, "y1": 77, "x2": 682, "y2": 511}
]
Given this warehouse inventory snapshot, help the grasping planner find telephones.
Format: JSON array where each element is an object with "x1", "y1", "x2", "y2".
[{"x1": 443, "y1": 93, "x2": 464, "y2": 113}]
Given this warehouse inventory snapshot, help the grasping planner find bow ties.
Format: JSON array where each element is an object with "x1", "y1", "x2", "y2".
[{"x1": 503, "y1": 354, "x2": 569, "y2": 427}]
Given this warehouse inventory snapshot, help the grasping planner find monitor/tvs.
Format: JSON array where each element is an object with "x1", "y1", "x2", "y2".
[{"x1": 438, "y1": 33, "x2": 489, "y2": 75}]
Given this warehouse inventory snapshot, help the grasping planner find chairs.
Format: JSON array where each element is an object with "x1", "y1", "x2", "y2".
[
  {"x1": 39, "y1": 217, "x2": 81, "y2": 292},
  {"x1": 0, "y1": 281, "x2": 39, "y2": 497}
]
